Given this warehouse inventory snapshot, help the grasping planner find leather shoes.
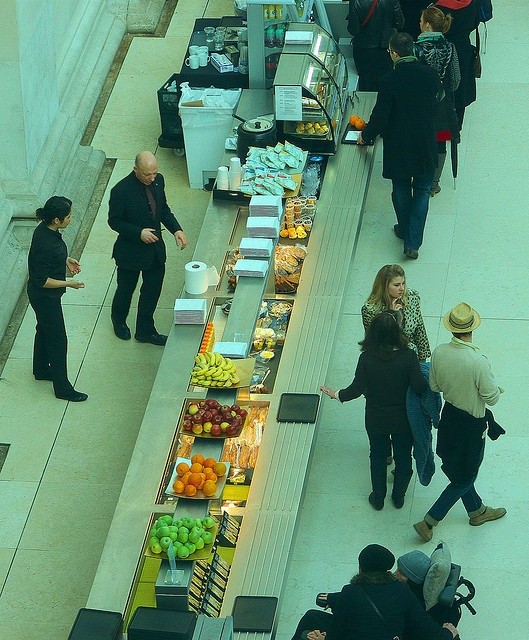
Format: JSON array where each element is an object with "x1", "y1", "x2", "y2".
[
  {"x1": 469, "y1": 505, "x2": 507, "y2": 526},
  {"x1": 34, "y1": 374, "x2": 54, "y2": 381},
  {"x1": 413, "y1": 520, "x2": 434, "y2": 542},
  {"x1": 369, "y1": 492, "x2": 385, "y2": 509},
  {"x1": 403, "y1": 246, "x2": 419, "y2": 258},
  {"x1": 55, "y1": 388, "x2": 88, "y2": 401},
  {"x1": 394, "y1": 224, "x2": 407, "y2": 240},
  {"x1": 134, "y1": 332, "x2": 167, "y2": 346},
  {"x1": 390, "y1": 493, "x2": 404, "y2": 509},
  {"x1": 111, "y1": 317, "x2": 131, "y2": 340}
]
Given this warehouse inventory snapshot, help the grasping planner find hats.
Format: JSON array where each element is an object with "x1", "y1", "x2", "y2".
[
  {"x1": 356, "y1": 543, "x2": 395, "y2": 573},
  {"x1": 398, "y1": 549, "x2": 431, "y2": 585},
  {"x1": 443, "y1": 302, "x2": 482, "y2": 333}
]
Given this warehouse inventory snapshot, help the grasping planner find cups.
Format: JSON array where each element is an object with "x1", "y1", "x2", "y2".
[
  {"x1": 212, "y1": 35, "x2": 225, "y2": 51},
  {"x1": 229, "y1": 157, "x2": 242, "y2": 196},
  {"x1": 204, "y1": 26, "x2": 214, "y2": 43},
  {"x1": 215, "y1": 26, "x2": 226, "y2": 33},
  {"x1": 196, "y1": 50, "x2": 206, "y2": 56},
  {"x1": 198, "y1": 45, "x2": 209, "y2": 53},
  {"x1": 216, "y1": 166, "x2": 229, "y2": 190},
  {"x1": 185, "y1": 54, "x2": 199, "y2": 70},
  {"x1": 214, "y1": 31, "x2": 225, "y2": 37},
  {"x1": 189, "y1": 45, "x2": 199, "y2": 56},
  {"x1": 198, "y1": 53, "x2": 211, "y2": 68},
  {"x1": 283, "y1": 195, "x2": 317, "y2": 232}
]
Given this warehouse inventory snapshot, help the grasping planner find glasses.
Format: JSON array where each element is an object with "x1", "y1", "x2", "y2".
[{"x1": 386, "y1": 48, "x2": 392, "y2": 52}]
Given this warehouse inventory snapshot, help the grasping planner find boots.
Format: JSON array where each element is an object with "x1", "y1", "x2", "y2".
[{"x1": 430, "y1": 151, "x2": 446, "y2": 198}]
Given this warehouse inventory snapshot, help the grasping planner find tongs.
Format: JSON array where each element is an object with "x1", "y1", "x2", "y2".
[{"x1": 228, "y1": 136, "x2": 238, "y2": 148}]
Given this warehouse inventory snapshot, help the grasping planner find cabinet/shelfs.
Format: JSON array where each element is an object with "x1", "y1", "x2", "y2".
[
  {"x1": 248, "y1": 1, "x2": 334, "y2": 89},
  {"x1": 273, "y1": 22, "x2": 347, "y2": 156}
]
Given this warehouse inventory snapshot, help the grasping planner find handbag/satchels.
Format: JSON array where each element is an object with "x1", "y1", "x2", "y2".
[{"x1": 472, "y1": 44, "x2": 481, "y2": 79}]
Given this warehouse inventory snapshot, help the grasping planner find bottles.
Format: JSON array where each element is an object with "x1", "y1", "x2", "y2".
[
  {"x1": 273, "y1": 53, "x2": 281, "y2": 68},
  {"x1": 309, "y1": 9, "x2": 315, "y2": 24},
  {"x1": 265, "y1": 56, "x2": 275, "y2": 79}
]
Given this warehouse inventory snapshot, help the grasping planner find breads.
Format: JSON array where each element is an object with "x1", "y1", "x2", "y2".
[{"x1": 228, "y1": 246, "x2": 306, "y2": 292}]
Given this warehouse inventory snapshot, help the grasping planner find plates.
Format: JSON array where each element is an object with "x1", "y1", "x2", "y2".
[
  {"x1": 187, "y1": 357, "x2": 257, "y2": 389},
  {"x1": 248, "y1": 145, "x2": 309, "y2": 174},
  {"x1": 178, "y1": 401, "x2": 248, "y2": 439},
  {"x1": 163, "y1": 455, "x2": 232, "y2": 500},
  {"x1": 244, "y1": 173, "x2": 304, "y2": 199},
  {"x1": 142, "y1": 514, "x2": 219, "y2": 561}
]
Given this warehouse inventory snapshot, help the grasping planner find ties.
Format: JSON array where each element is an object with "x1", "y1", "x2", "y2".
[{"x1": 146, "y1": 186, "x2": 157, "y2": 220}]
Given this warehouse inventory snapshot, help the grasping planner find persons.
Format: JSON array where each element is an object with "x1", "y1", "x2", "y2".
[
  {"x1": 357, "y1": 31, "x2": 442, "y2": 260},
  {"x1": 345, "y1": 0, "x2": 405, "y2": 91},
  {"x1": 107, "y1": 150, "x2": 187, "y2": 346},
  {"x1": 419, "y1": 0, "x2": 493, "y2": 130},
  {"x1": 412, "y1": 6, "x2": 461, "y2": 196},
  {"x1": 412, "y1": 301, "x2": 507, "y2": 542},
  {"x1": 290, "y1": 550, "x2": 430, "y2": 640},
  {"x1": 320, "y1": 310, "x2": 428, "y2": 511},
  {"x1": 309, "y1": 543, "x2": 458, "y2": 640},
  {"x1": 26, "y1": 196, "x2": 88, "y2": 401},
  {"x1": 361, "y1": 263, "x2": 431, "y2": 476}
]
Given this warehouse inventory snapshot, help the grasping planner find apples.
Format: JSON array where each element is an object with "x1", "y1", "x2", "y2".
[
  {"x1": 182, "y1": 399, "x2": 248, "y2": 435},
  {"x1": 148, "y1": 515, "x2": 215, "y2": 559}
]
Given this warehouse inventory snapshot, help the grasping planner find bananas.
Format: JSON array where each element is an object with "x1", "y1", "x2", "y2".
[{"x1": 190, "y1": 353, "x2": 239, "y2": 388}]
